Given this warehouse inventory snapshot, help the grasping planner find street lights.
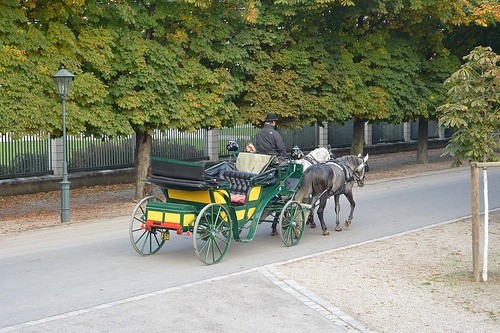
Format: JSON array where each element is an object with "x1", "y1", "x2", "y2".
[{"x1": 51, "y1": 65, "x2": 76, "y2": 224}]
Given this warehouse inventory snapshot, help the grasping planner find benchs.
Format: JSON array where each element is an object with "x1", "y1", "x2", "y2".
[{"x1": 217, "y1": 170, "x2": 257, "y2": 205}]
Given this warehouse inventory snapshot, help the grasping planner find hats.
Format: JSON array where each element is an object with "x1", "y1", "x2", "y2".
[{"x1": 264, "y1": 113, "x2": 278, "y2": 121}]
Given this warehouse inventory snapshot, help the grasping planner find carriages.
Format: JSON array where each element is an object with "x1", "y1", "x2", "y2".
[{"x1": 129, "y1": 139, "x2": 370, "y2": 266}]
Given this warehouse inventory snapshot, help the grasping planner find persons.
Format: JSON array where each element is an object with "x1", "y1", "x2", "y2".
[{"x1": 255, "y1": 112, "x2": 287, "y2": 159}]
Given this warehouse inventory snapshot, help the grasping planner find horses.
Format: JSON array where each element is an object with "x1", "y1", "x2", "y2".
[
  {"x1": 295, "y1": 153, "x2": 370, "y2": 235},
  {"x1": 271, "y1": 144, "x2": 332, "y2": 235}
]
